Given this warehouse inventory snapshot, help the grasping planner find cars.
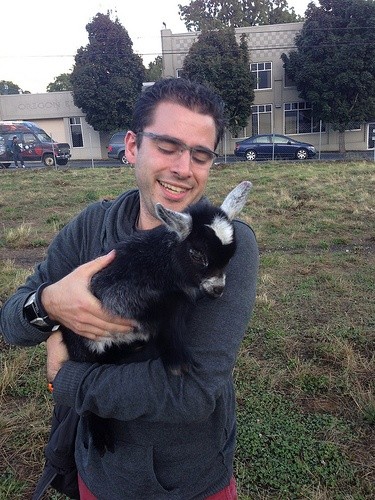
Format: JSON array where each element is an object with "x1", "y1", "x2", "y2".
[
  {"x1": 107, "y1": 128, "x2": 135, "y2": 164},
  {"x1": 232, "y1": 132, "x2": 319, "y2": 162}
]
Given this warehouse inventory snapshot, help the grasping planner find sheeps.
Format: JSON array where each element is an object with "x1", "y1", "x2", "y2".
[{"x1": 60, "y1": 178, "x2": 253, "y2": 458}]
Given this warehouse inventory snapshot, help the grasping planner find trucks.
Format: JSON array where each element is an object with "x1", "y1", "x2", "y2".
[{"x1": 0, "y1": 118, "x2": 72, "y2": 169}]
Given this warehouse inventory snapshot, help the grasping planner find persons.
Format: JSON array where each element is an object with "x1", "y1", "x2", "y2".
[
  {"x1": 11, "y1": 136, "x2": 26, "y2": 168},
  {"x1": 0, "y1": 77, "x2": 258, "y2": 500}
]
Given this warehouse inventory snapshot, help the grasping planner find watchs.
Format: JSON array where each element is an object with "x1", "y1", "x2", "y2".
[{"x1": 25, "y1": 292, "x2": 48, "y2": 327}]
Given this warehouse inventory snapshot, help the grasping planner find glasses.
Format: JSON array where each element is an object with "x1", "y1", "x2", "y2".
[{"x1": 137, "y1": 131, "x2": 218, "y2": 168}]
